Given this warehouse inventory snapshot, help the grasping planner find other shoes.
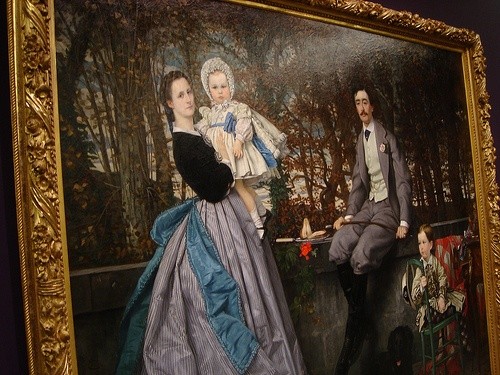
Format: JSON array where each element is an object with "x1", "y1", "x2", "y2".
[
  {"x1": 256, "y1": 225, "x2": 269, "y2": 243},
  {"x1": 259, "y1": 209, "x2": 273, "y2": 225}
]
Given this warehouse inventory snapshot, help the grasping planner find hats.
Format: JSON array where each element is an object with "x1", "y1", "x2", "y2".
[{"x1": 201, "y1": 56, "x2": 235, "y2": 111}]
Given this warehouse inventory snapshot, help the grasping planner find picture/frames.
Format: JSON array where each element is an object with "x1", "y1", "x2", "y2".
[{"x1": 4, "y1": 0, "x2": 500, "y2": 375}]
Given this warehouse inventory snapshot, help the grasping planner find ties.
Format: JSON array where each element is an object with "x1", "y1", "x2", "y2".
[{"x1": 365, "y1": 130, "x2": 371, "y2": 141}]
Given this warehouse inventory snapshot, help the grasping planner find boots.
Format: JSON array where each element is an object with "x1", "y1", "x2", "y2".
[{"x1": 335, "y1": 261, "x2": 369, "y2": 375}]
